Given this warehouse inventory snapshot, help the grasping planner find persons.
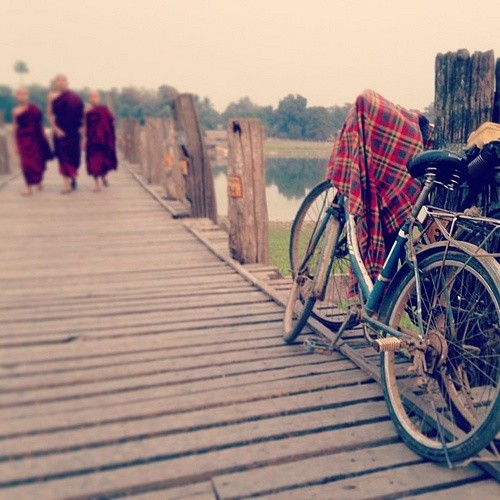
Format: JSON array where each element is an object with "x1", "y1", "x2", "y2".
[
  {"x1": 80, "y1": 89, "x2": 119, "y2": 193},
  {"x1": 11, "y1": 86, "x2": 54, "y2": 196},
  {"x1": 45, "y1": 74, "x2": 86, "y2": 194}
]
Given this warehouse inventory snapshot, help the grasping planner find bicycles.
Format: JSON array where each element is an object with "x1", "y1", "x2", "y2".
[{"x1": 282, "y1": 88, "x2": 500, "y2": 475}]
